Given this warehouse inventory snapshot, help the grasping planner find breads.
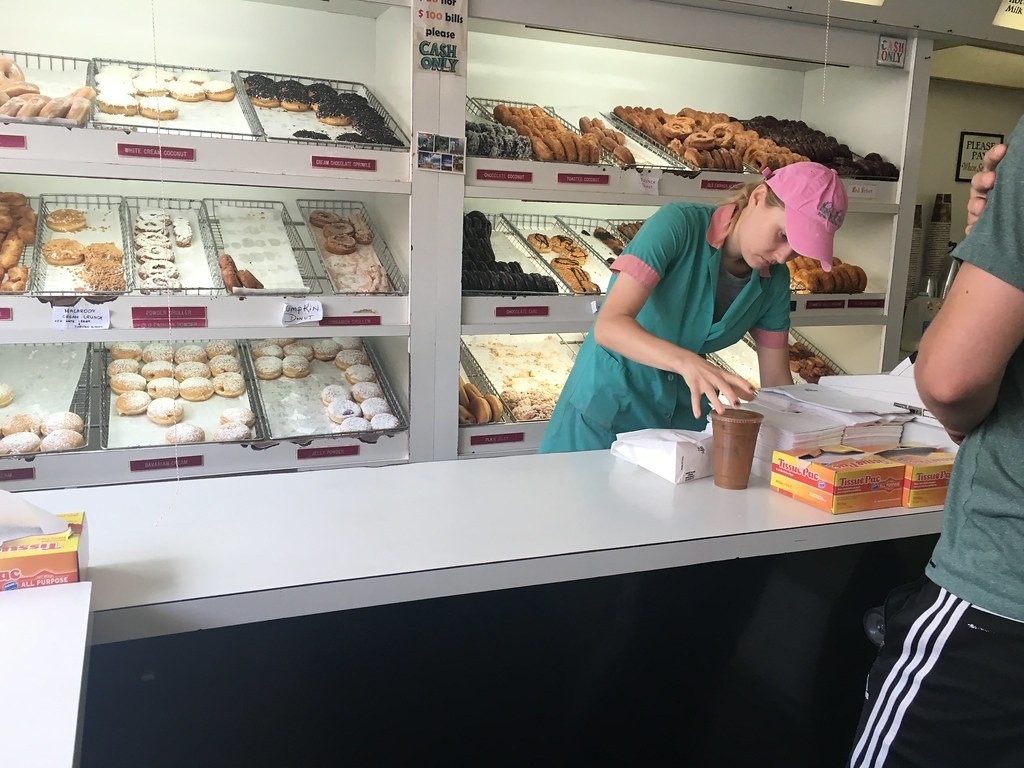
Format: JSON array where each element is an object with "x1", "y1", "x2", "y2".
[
  {"x1": 459, "y1": 337, "x2": 842, "y2": 425},
  {"x1": 461, "y1": 209, "x2": 868, "y2": 298},
  {"x1": 1, "y1": 48, "x2": 405, "y2": 459}
]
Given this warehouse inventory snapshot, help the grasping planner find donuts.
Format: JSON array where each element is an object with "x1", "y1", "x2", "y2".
[{"x1": 466, "y1": 104, "x2": 901, "y2": 182}]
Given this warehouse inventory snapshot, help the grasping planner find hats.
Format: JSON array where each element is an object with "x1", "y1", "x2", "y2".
[{"x1": 760, "y1": 160, "x2": 848, "y2": 272}]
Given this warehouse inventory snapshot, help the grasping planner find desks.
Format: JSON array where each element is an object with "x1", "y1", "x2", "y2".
[
  {"x1": 1, "y1": 446, "x2": 1024, "y2": 768},
  {"x1": 0, "y1": 581, "x2": 93, "y2": 767}
]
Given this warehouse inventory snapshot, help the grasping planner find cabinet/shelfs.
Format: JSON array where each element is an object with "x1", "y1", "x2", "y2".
[
  {"x1": 0, "y1": 1, "x2": 442, "y2": 478},
  {"x1": 433, "y1": 1, "x2": 933, "y2": 463}
]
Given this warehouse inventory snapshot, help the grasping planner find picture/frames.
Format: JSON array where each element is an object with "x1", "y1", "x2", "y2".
[{"x1": 955, "y1": 131, "x2": 1004, "y2": 184}]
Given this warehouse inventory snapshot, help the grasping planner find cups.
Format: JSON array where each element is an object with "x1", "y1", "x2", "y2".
[{"x1": 709, "y1": 408, "x2": 764, "y2": 491}]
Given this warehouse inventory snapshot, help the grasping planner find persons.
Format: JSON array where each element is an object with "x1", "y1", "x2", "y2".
[
  {"x1": 538, "y1": 157, "x2": 847, "y2": 457},
  {"x1": 845, "y1": 116, "x2": 1024, "y2": 768}
]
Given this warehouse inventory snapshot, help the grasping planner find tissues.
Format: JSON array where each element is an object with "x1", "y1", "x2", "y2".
[
  {"x1": 858, "y1": 442, "x2": 964, "y2": 511},
  {"x1": 0, "y1": 489, "x2": 93, "y2": 601},
  {"x1": 770, "y1": 442, "x2": 906, "y2": 518}
]
